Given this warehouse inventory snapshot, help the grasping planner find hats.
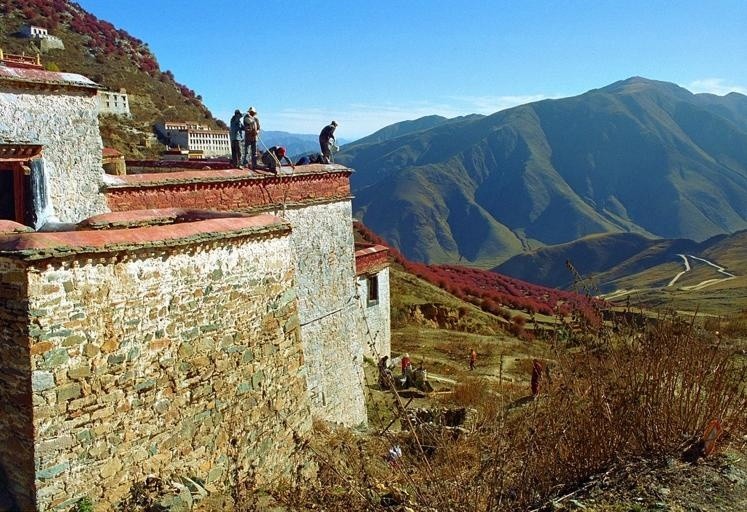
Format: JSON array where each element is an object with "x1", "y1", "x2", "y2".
[
  {"x1": 331, "y1": 120, "x2": 339, "y2": 125},
  {"x1": 277, "y1": 148, "x2": 284, "y2": 156},
  {"x1": 246, "y1": 107, "x2": 257, "y2": 114}
]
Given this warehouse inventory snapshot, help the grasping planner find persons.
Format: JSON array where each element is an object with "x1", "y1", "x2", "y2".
[
  {"x1": 261, "y1": 145, "x2": 295, "y2": 174},
  {"x1": 402, "y1": 352, "x2": 412, "y2": 377},
  {"x1": 241, "y1": 105, "x2": 260, "y2": 168},
  {"x1": 295, "y1": 153, "x2": 317, "y2": 166},
  {"x1": 228, "y1": 108, "x2": 245, "y2": 168},
  {"x1": 318, "y1": 120, "x2": 339, "y2": 162},
  {"x1": 378, "y1": 356, "x2": 389, "y2": 382},
  {"x1": 469, "y1": 348, "x2": 478, "y2": 372},
  {"x1": 529, "y1": 358, "x2": 543, "y2": 397}
]
